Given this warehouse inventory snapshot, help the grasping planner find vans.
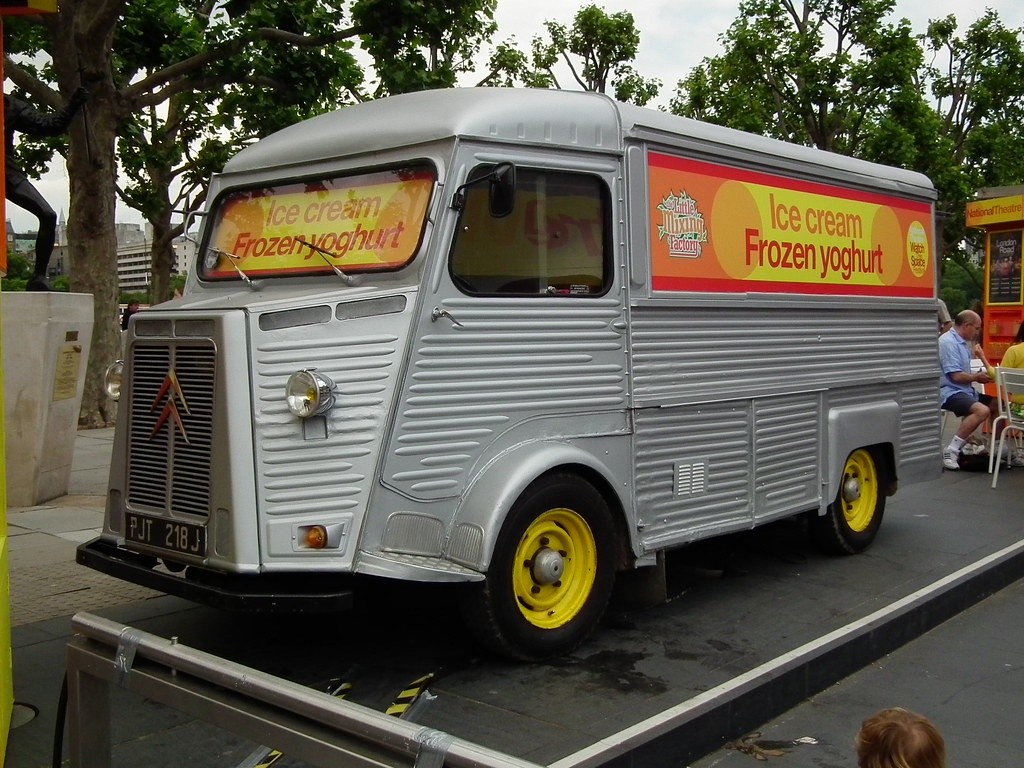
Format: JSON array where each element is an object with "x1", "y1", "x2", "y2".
[{"x1": 72, "y1": 85, "x2": 946, "y2": 662}]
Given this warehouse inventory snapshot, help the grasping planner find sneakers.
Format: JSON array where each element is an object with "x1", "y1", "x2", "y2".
[
  {"x1": 994, "y1": 450, "x2": 1024, "y2": 465},
  {"x1": 942, "y1": 447, "x2": 959, "y2": 469}
]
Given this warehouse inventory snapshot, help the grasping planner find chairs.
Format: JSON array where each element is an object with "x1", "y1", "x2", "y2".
[{"x1": 940, "y1": 367, "x2": 1024, "y2": 488}]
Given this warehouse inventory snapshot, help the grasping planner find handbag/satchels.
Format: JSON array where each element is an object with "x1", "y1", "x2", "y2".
[{"x1": 958, "y1": 442, "x2": 995, "y2": 473}]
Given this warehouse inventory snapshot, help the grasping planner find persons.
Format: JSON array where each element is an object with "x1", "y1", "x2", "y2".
[
  {"x1": 3, "y1": 50, "x2": 89, "y2": 292},
  {"x1": 855, "y1": 706, "x2": 946, "y2": 768},
  {"x1": 975, "y1": 318, "x2": 1024, "y2": 464},
  {"x1": 122, "y1": 300, "x2": 139, "y2": 332},
  {"x1": 938, "y1": 298, "x2": 1024, "y2": 469}
]
